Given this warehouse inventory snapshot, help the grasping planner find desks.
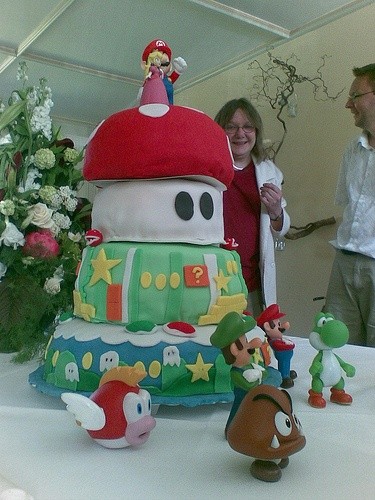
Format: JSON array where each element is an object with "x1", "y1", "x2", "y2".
[{"x1": 1, "y1": 335, "x2": 374, "y2": 500}]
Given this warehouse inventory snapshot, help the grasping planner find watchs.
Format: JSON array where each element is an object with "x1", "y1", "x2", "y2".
[{"x1": 271, "y1": 212, "x2": 283, "y2": 221}]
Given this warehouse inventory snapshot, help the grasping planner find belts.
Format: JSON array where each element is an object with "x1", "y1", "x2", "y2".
[{"x1": 337, "y1": 249, "x2": 375, "y2": 260}]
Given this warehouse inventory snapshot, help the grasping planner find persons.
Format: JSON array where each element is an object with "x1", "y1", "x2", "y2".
[
  {"x1": 327, "y1": 63, "x2": 375, "y2": 348},
  {"x1": 210, "y1": 96, "x2": 290, "y2": 324},
  {"x1": 256, "y1": 304, "x2": 298, "y2": 388},
  {"x1": 136, "y1": 40, "x2": 188, "y2": 105},
  {"x1": 212, "y1": 311, "x2": 282, "y2": 439}
]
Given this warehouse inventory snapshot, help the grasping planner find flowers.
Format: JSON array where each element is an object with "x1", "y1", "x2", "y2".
[{"x1": 0, "y1": 47, "x2": 93, "y2": 362}]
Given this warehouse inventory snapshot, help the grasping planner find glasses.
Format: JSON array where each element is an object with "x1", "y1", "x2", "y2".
[
  {"x1": 349, "y1": 91, "x2": 375, "y2": 103},
  {"x1": 225, "y1": 121, "x2": 256, "y2": 133}
]
